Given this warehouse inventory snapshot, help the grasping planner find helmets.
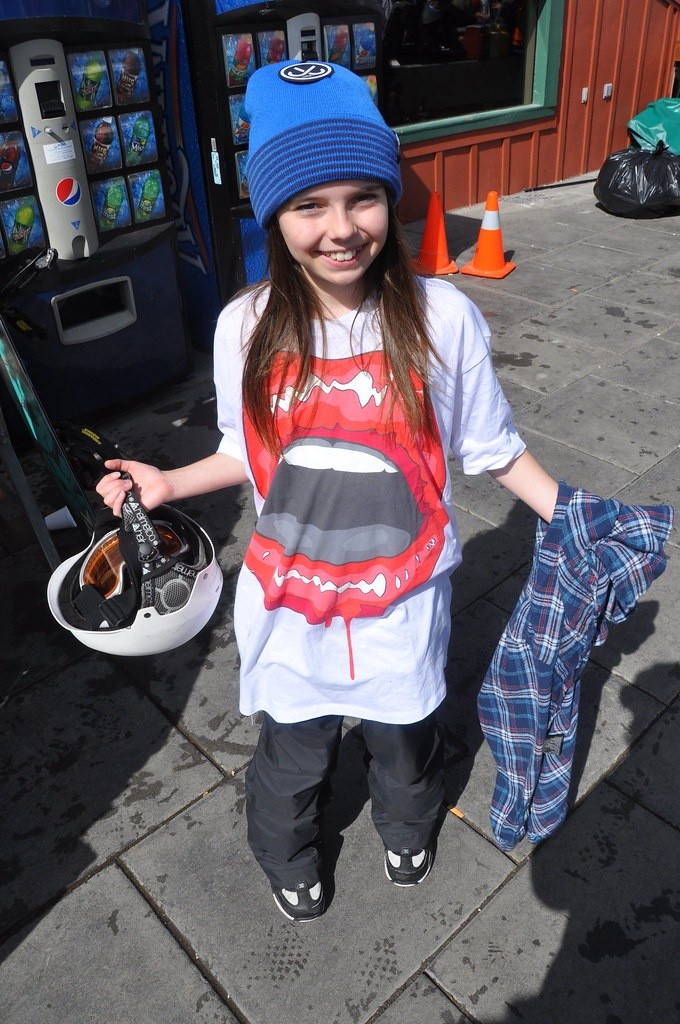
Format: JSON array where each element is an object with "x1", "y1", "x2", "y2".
[{"x1": 47, "y1": 446, "x2": 224, "y2": 655}]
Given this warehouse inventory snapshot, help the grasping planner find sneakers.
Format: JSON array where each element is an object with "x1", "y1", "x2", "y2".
[
  {"x1": 383, "y1": 843, "x2": 433, "y2": 887},
  {"x1": 271, "y1": 877, "x2": 325, "y2": 923}
]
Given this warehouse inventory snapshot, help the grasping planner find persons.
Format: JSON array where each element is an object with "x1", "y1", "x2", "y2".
[{"x1": 95, "y1": 60, "x2": 673, "y2": 923}]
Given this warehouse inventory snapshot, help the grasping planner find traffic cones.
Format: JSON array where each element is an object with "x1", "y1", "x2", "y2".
[
  {"x1": 409, "y1": 193, "x2": 459, "y2": 275},
  {"x1": 461, "y1": 191, "x2": 516, "y2": 278},
  {"x1": 512, "y1": 28, "x2": 524, "y2": 50}
]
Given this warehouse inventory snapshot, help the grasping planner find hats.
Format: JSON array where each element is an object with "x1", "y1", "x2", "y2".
[{"x1": 245, "y1": 60, "x2": 402, "y2": 231}]
